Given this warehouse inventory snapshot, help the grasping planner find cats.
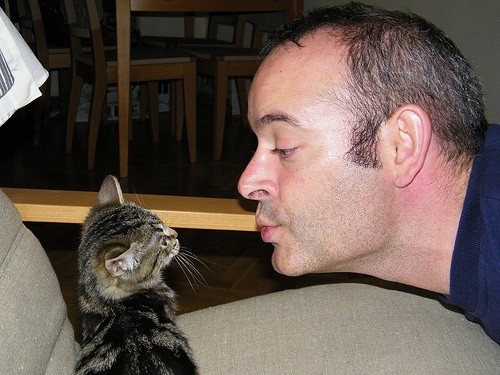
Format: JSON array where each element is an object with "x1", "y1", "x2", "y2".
[{"x1": 73, "y1": 174, "x2": 217, "y2": 375}]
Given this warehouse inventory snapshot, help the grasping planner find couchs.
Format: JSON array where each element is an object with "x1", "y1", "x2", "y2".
[{"x1": 0, "y1": 191, "x2": 500, "y2": 374}]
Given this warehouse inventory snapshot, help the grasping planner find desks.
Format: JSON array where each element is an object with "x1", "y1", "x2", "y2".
[{"x1": 100, "y1": 2, "x2": 305, "y2": 180}]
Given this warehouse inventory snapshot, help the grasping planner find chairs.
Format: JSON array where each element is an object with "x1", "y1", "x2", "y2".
[
  {"x1": 65, "y1": 1, "x2": 197, "y2": 179},
  {"x1": 0, "y1": 0, "x2": 161, "y2": 147},
  {"x1": 161, "y1": 1, "x2": 305, "y2": 162},
  {"x1": 138, "y1": 12, "x2": 251, "y2": 132}
]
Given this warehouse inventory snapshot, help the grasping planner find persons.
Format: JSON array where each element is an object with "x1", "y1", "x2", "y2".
[{"x1": 236, "y1": 0, "x2": 500, "y2": 349}]
